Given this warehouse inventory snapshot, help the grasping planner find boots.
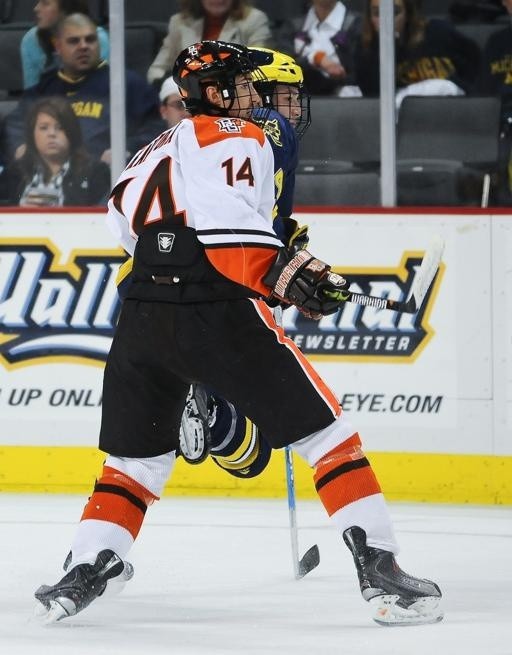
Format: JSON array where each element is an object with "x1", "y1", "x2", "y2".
[
  {"x1": 34, "y1": 545, "x2": 125, "y2": 617},
  {"x1": 342, "y1": 525, "x2": 442, "y2": 603}
]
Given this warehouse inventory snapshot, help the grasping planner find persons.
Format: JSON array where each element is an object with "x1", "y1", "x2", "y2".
[
  {"x1": 0, "y1": 0, "x2": 512, "y2": 210},
  {"x1": 176, "y1": 47, "x2": 350, "y2": 478},
  {"x1": 32, "y1": 40, "x2": 441, "y2": 608}
]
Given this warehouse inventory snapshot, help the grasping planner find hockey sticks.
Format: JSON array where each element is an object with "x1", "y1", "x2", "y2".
[
  {"x1": 320, "y1": 238, "x2": 443, "y2": 313},
  {"x1": 285, "y1": 438, "x2": 321, "y2": 581}
]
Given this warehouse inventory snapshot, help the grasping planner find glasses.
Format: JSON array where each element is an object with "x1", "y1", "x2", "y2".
[{"x1": 165, "y1": 99, "x2": 184, "y2": 112}]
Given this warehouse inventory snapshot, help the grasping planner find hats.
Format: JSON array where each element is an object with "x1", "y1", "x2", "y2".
[{"x1": 158, "y1": 75, "x2": 182, "y2": 103}]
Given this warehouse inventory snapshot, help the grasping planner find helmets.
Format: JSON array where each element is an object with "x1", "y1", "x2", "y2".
[
  {"x1": 247, "y1": 44, "x2": 312, "y2": 142},
  {"x1": 172, "y1": 39, "x2": 273, "y2": 129}
]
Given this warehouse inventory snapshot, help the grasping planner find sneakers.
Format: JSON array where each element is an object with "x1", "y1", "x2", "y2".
[{"x1": 177, "y1": 383, "x2": 214, "y2": 466}]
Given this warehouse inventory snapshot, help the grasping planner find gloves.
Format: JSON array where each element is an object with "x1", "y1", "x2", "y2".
[{"x1": 262, "y1": 244, "x2": 352, "y2": 323}]
